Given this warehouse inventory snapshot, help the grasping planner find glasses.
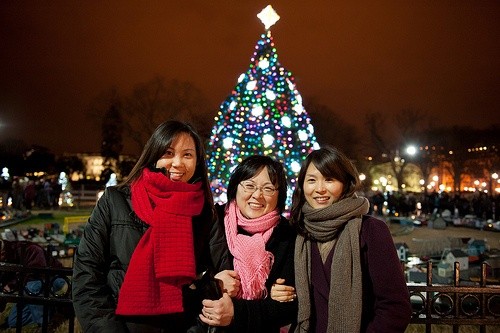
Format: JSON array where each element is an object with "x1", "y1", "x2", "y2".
[{"x1": 239, "y1": 181, "x2": 279, "y2": 195}]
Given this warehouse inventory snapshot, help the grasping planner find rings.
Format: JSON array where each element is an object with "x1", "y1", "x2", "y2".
[{"x1": 207, "y1": 316, "x2": 212, "y2": 320}]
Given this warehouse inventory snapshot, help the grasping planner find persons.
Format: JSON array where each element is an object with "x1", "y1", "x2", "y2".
[
  {"x1": 72, "y1": 121, "x2": 231, "y2": 333},
  {"x1": 365, "y1": 189, "x2": 500, "y2": 223},
  {"x1": 0, "y1": 168, "x2": 120, "y2": 333},
  {"x1": 204, "y1": 155, "x2": 301, "y2": 333},
  {"x1": 269, "y1": 147, "x2": 413, "y2": 333}
]
